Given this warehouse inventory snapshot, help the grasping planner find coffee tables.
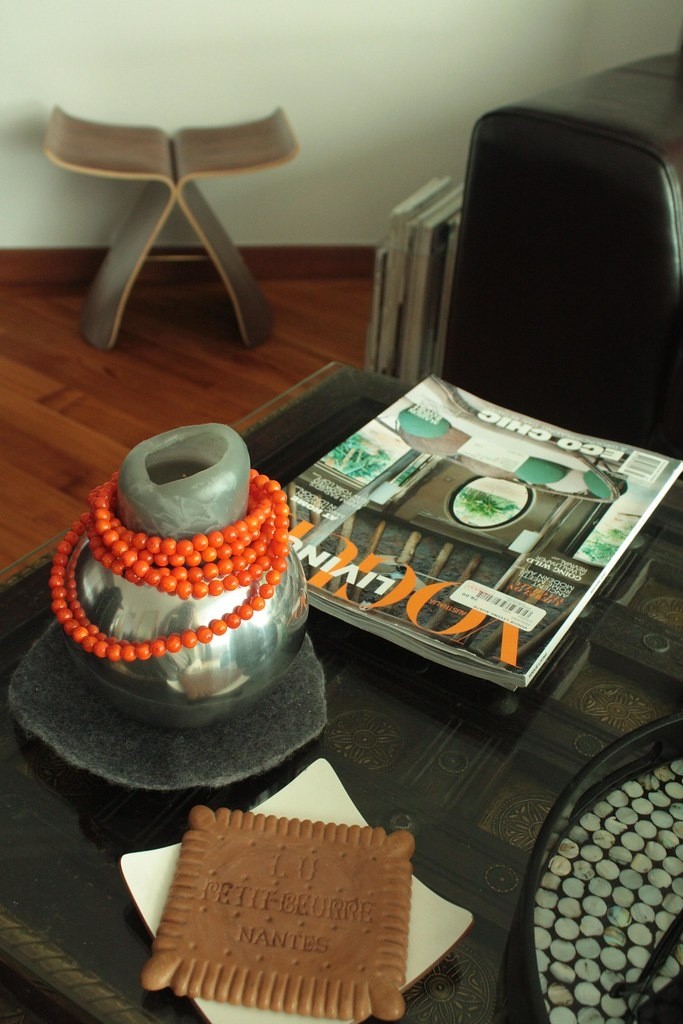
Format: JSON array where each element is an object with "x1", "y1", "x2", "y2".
[{"x1": 0, "y1": 361, "x2": 683, "y2": 1024}]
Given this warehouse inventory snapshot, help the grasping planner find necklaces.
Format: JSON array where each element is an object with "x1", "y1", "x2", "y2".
[{"x1": 44, "y1": 461, "x2": 291, "y2": 660}]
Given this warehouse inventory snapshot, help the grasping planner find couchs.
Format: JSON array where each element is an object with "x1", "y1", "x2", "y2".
[{"x1": 441, "y1": 52, "x2": 683, "y2": 479}]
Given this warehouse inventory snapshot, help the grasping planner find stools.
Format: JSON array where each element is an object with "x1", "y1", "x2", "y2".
[{"x1": 40, "y1": 103, "x2": 301, "y2": 347}]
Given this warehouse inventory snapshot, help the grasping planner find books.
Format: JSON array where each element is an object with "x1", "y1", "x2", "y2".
[
  {"x1": 366, "y1": 174, "x2": 464, "y2": 382},
  {"x1": 282, "y1": 374, "x2": 683, "y2": 693}
]
